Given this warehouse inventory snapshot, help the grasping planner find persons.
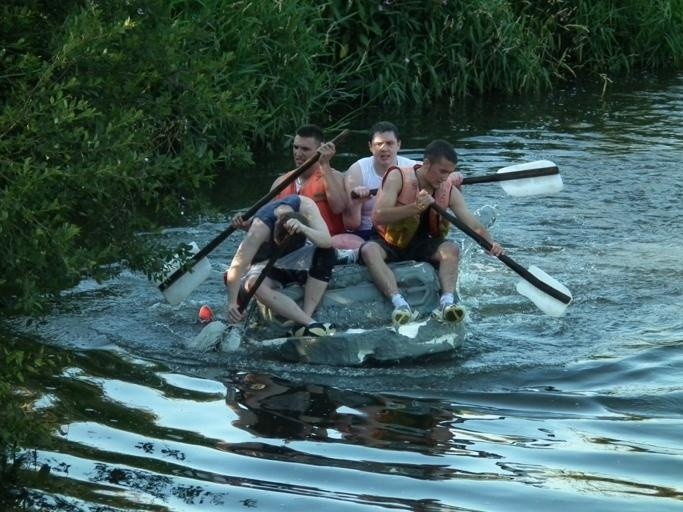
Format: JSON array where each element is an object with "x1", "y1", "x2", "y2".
[
  {"x1": 226, "y1": 195, "x2": 337, "y2": 337},
  {"x1": 231, "y1": 124, "x2": 349, "y2": 238},
  {"x1": 341, "y1": 120, "x2": 463, "y2": 240},
  {"x1": 359, "y1": 139, "x2": 503, "y2": 327}
]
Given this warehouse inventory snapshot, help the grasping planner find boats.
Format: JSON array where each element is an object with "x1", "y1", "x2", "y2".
[{"x1": 243, "y1": 258, "x2": 466, "y2": 368}]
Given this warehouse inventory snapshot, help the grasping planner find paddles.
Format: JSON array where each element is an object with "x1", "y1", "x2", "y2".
[
  {"x1": 150, "y1": 128, "x2": 350, "y2": 305},
  {"x1": 351, "y1": 159, "x2": 563, "y2": 201},
  {"x1": 188, "y1": 231, "x2": 290, "y2": 353},
  {"x1": 428, "y1": 204, "x2": 572, "y2": 317}
]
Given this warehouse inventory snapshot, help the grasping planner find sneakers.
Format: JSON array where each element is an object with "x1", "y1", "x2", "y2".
[
  {"x1": 430, "y1": 303, "x2": 466, "y2": 326},
  {"x1": 389, "y1": 302, "x2": 420, "y2": 327},
  {"x1": 281, "y1": 321, "x2": 336, "y2": 339}
]
